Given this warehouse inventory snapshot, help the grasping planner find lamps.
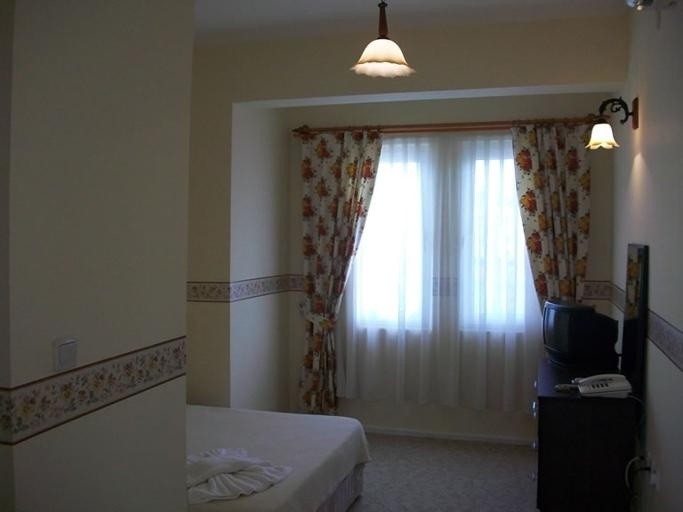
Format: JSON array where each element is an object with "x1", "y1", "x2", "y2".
[
  {"x1": 585, "y1": 95, "x2": 638, "y2": 150},
  {"x1": 347, "y1": 0, "x2": 416, "y2": 79}
]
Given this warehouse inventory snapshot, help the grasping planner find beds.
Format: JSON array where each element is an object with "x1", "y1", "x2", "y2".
[{"x1": 184, "y1": 406, "x2": 369, "y2": 512}]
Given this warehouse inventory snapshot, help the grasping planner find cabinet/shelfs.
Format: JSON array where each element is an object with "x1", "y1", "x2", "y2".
[{"x1": 531, "y1": 357, "x2": 643, "y2": 512}]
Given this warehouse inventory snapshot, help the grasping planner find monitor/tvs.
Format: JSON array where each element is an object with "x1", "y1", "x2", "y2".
[{"x1": 542, "y1": 301, "x2": 618, "y2": 371}]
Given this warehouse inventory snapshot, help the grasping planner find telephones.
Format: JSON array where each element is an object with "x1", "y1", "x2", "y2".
[{"x1": 579, "y1": 374, "x2": 631, "y2": 399}]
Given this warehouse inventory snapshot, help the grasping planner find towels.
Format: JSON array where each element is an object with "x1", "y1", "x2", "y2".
[{"x1": 184, "y1": 446, "x2": 293, "y2": 505}]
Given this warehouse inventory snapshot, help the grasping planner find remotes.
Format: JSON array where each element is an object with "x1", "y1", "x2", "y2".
[{"x1": 555, "y1": 383, "x2": 578, "y2": 392}]
